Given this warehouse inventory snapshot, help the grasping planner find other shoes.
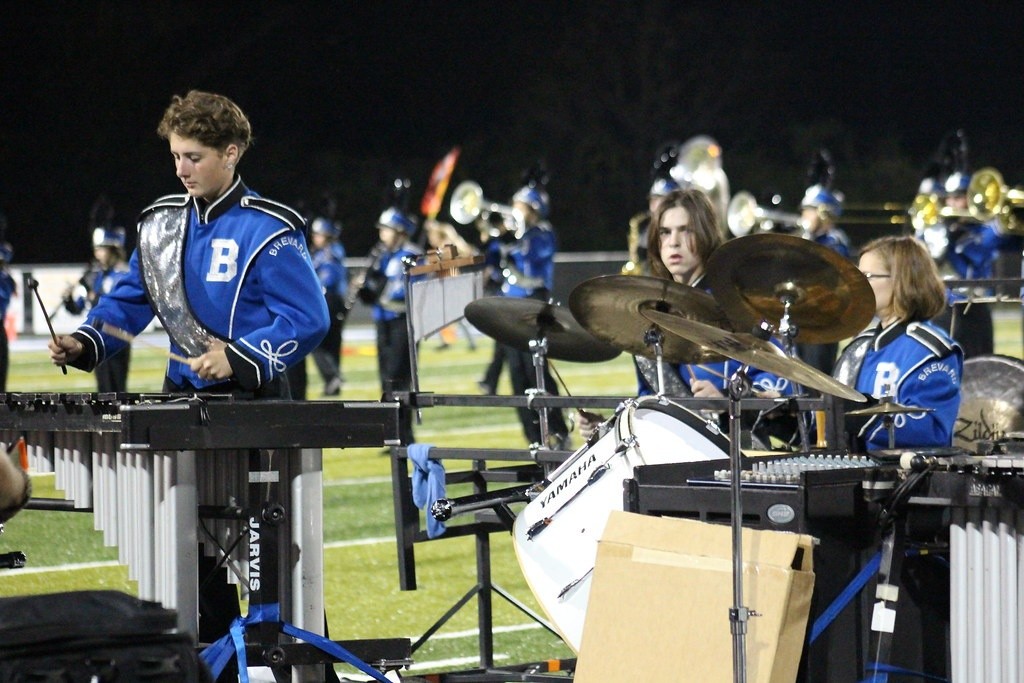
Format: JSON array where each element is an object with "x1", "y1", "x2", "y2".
[
  {"x1": 324, "y1": 378, "x2": 341, "y2": 396},
  {"x1": 478, "y1": 380, "x2": 496, "y2": 395},
  {"x1": 554, "y1": 437, "x2": 572, "y2": 451}
]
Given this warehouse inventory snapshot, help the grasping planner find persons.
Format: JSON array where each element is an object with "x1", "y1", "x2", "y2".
[
  {"x1": 793, "y1": 182, "x2": 850, "y2": 258},
  {"x1": 359, "y1": 177, "x2": 416, "y2": 453},
  {"x1": 909, "y1": 170, "x2": 995, "y2": 358},
  {"x1": 638, "y1": 189, "x2": 803, "y2": 402},
  {"x1": 628, "y1": 176, "x2": 670, "y2": 277},
  {"x1": 829, "y1": 234, "x2": 963, "y2": 451},
  {"x1": 0, "y1": 240, "x2": 17, "y2": 395},
  {"x1": 479, "y1": 161, "x2": 568, "y2": 444},
  {"x1": 303, "y1": 216, "x2": 351, "y2": 392},
  {"x1": 49, "y1": 91, "x2": 331, "y2": 401},
  {"x1": 65, "y1": 225, "x2": 134, "y2": 391},
  {"x1": 0, "y1": 409, "x2": 33, "y2": 522}
]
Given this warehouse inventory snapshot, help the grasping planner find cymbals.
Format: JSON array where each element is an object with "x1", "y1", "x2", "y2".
[
  {"x1": 567, "y1": 272, "x2": 741, "y2": 366},
  {"x1": 705, "y1": 230, "x2": 878, "y2": 347},
  {"x1": 639, "y1": 306, "x2": 869, "y2": 404},
  {"x1": 463, "y1": 296, "x2": 624, "y2": 364},
  {"x1": 836, "y1": 399, "x2": 937, "y2": 416}
]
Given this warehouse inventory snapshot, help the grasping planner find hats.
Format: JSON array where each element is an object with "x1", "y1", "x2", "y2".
[
  {"x1": 919, "y1": 175, "x2": 947, "y2": 199},
  {"x1": 650, "y1": 177, "x2": 676, "y2": 198},
  {"x1": 0, "y1": 240, "x2": 14, "y2": 263},
  {"x1": 801, "y1": 184, "x2": 841, "y2": 221},
  {"x1": 311, "y1": 214, "x2": 342, "y2": 239},
  {"x1": 374, "y1": 206, "x2": 421, "y2": 241},
  {"x1": 943, "y1": 171, "x2": 969, "y2": 196},
  {"x1": 91, "y1": 226, "x2": 126, "y2": 249},
  {"x1": 512, "y1": 185, "x2": 553, "y2": 220}
]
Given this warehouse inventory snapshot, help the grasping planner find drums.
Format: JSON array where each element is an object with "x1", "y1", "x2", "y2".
[
  {"x1": 509, "y1": 391, "x2": 750, "y2": 661},
  {"x1": 890, "y1": 351, "x2": 1024, "y2": 454}
]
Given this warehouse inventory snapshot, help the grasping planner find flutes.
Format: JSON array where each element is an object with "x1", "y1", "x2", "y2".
[{"x1": 336, "y1": 239, "x2": 383, "y2": 322}]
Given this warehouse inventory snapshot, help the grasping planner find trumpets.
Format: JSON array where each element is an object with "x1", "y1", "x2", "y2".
[
  {"x1": 726, "y1": 191, "x2": 808, "y2": 237},
  {"x1": 448, "y1": 179, "x2": 527, "y2": 238},
  {"x1": 966, "y1": 166, "x2": 1024, "y2": 237}
]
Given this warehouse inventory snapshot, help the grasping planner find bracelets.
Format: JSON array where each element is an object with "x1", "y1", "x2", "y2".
[{"x1": 20, "y1": 463, "x2": 31, "y2": 508}]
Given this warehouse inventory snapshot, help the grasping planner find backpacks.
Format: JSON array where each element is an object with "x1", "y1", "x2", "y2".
[{"x1": 0, "y1": 591, "x2": 215, "y2": 683}]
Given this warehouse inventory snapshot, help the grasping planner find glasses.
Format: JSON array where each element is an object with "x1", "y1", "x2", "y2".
[{"x1": 863, "y1": 271, "x2": 890, "y2": 284}]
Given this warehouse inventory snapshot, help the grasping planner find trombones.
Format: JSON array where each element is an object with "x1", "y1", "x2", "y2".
[{"x1": 822, "y1": 196, "x2": 975, "y2": 230}]
